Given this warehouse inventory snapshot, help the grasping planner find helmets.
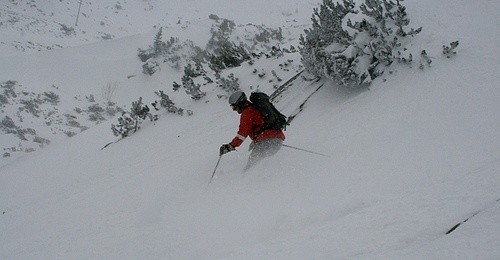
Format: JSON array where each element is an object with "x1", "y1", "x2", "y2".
[{"x1": 229, "y1": 91, "x2": 249, "y2": 112}]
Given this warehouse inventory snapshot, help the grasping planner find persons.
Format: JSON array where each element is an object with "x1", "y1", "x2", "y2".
[{"x1": 215, "y1": 89, "x2": 286, "y2": 181}]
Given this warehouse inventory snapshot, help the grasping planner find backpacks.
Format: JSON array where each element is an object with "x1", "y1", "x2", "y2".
[{"x1": 250, "y1": 92, "x2": 288, "y2": 140}]
